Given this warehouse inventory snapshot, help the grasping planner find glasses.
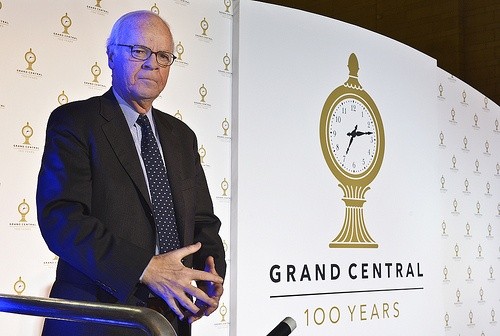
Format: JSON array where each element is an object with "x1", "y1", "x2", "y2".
[{"x1": 112, "y1": 43, "x2": 177, "y2": 66}]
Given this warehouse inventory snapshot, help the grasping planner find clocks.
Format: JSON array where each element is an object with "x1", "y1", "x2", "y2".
[{"x1": 320, "y1": 52, "x2": 386, "y2": 248}]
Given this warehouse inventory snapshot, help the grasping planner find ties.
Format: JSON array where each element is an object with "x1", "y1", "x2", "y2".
[{"x1": 133, "y1": 114, "x2": 181, "y2": 255}]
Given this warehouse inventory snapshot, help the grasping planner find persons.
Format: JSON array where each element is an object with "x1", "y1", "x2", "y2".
[{"x1": 36, "y1": 11, "x2": 227, "y2": 336}]
